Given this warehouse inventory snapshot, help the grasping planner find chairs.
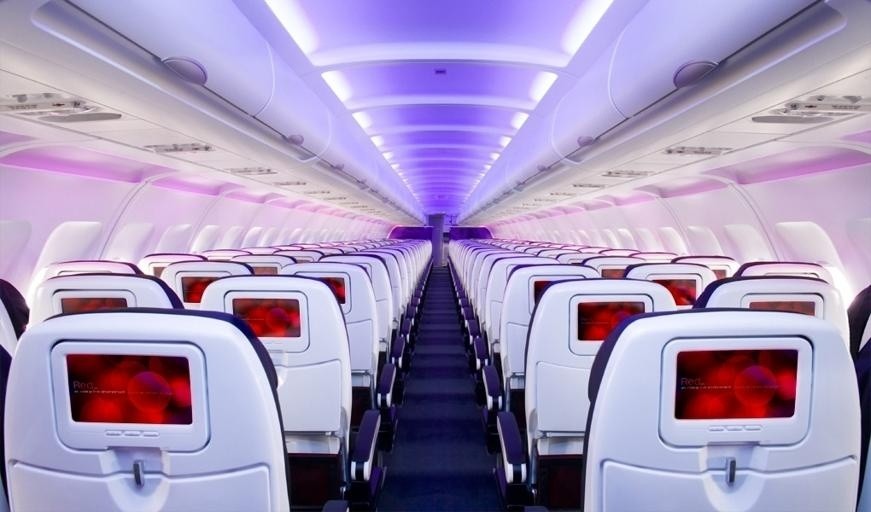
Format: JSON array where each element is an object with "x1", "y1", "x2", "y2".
[
  {"x1": 0, "y1": 238, "x2": 434, "y2": 512},
  {"x1": 447, "y1": 238, "x2": 871, "y2": 511}
]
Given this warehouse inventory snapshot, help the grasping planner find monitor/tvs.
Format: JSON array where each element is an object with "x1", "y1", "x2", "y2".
[
  {"x1": 50, "y1": 342, "x2": 209, "y2": 450},
  {"x1": 659, "y1": 336, "x2": 812, "y2": 446},
  {"x1": 149, "y1": 263, "x2": 170, "y2": 279},
  {"x1": 246, "y1": 263, "x2": 282, "y2": 276},
  {"x1": 176, "y1": 271, "x2": 232, "y2": 310},
  {"x1": 224, "y1": 291, "x2": 309, "y2": 353},
  {"x1": 296, "y1": 272, "x2": 351, "y2": 314},
  {"x1": 528, "y1": 263, "x2": 824, "y2": 320},
  {"x1": 569, "y1": 295, "x2": 653, "y2": 356},
  {"x1": 52, "y1": 291, "x2": 136, "y2": 315}
]
